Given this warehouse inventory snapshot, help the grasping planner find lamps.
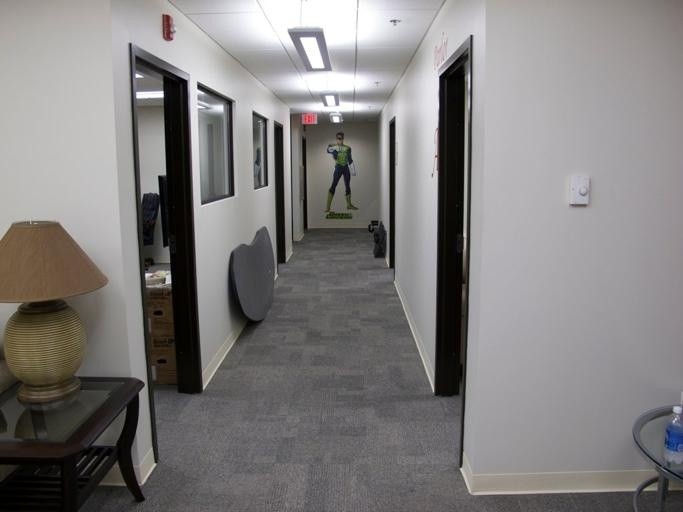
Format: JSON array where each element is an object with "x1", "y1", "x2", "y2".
[{"x1": 0, "y1": 220, "x2": 107, "y2": 402}]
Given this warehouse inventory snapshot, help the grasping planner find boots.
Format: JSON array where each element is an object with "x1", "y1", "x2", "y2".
[
  {"x1": 324, "y1": 192, "x2": 333, "y2": 211},
  {"x1": 346, "y1": 192, "x2": 357, "y2": 209}
]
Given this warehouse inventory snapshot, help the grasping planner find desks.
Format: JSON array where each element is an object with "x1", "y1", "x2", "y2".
[{"x1": 0, "y1": 376, "x2": 147, "y2": 512}]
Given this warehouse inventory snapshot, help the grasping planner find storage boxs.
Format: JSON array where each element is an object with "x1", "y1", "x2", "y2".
[{"x1": 144, "y1": 269, "x2": 179, "y2": 385}]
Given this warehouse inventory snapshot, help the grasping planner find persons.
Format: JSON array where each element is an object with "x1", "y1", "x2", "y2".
[{"x1": 325, "y1": 131, "x2": 359, "y2": 212}]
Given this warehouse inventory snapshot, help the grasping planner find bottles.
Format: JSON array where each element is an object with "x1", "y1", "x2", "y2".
[{"x1": 662, "y1": 406, "x2": 683, "y2": 465}]
